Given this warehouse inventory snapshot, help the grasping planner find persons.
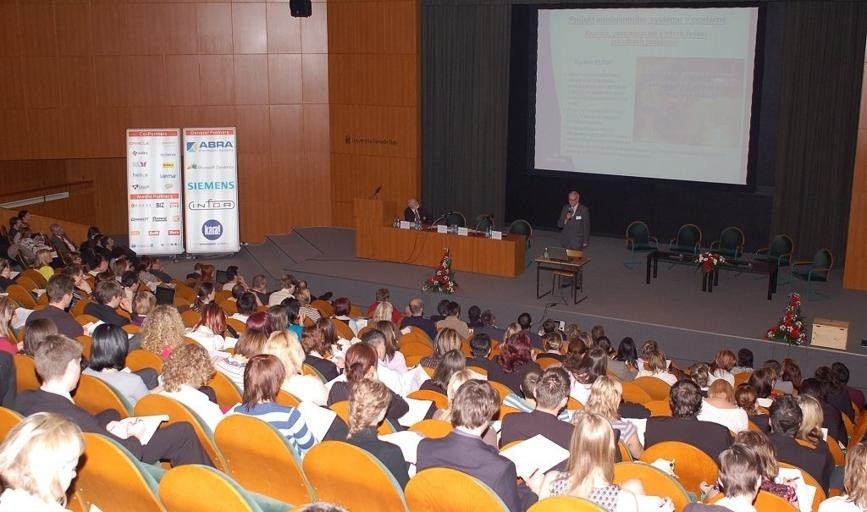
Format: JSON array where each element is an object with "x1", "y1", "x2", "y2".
[
  {"x1": 559, "y1": 192, "x2": 590, "y2": 287},
  {"x1": 406, "y1": 198, "x2": 433, "y2": 224},
  {"x1": 1, "y1": 210, "x2": 867, "y2": 512}
]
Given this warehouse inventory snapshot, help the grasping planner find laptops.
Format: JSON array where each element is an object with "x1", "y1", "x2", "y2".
[
  {"x1": 156, "y1": 286, "x2": 176, "y2": 305},
  {"x1": 548, "y1": 246, "x2": 571, "y2": 261}
]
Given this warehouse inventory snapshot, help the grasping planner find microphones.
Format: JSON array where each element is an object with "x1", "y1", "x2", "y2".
[
  {"x1": 567, "y1": 206, "x2": 573, "y2": 221},
  {"x1": 369, "y1": 186, "x2": 383, "y2": 199}
]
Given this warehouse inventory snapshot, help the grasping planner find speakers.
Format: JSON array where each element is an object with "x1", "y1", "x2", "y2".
[{"x1": 290, "y1": 2, "x2": 311, "y2": 17}]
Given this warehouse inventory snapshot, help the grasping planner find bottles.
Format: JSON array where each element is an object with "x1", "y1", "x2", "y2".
[
  {"x1": 475, "y1": 224, "x2": 492, "y2": 238},
  {"x1": 393, "y1": 218, "x2": 423, "y2": 231},
  {"x1": 454, "y1": 224, "x2": 457, "y2": 234},
  {"x1": 543, "y1": 247, "x2": 548, "y2": 260}
]
